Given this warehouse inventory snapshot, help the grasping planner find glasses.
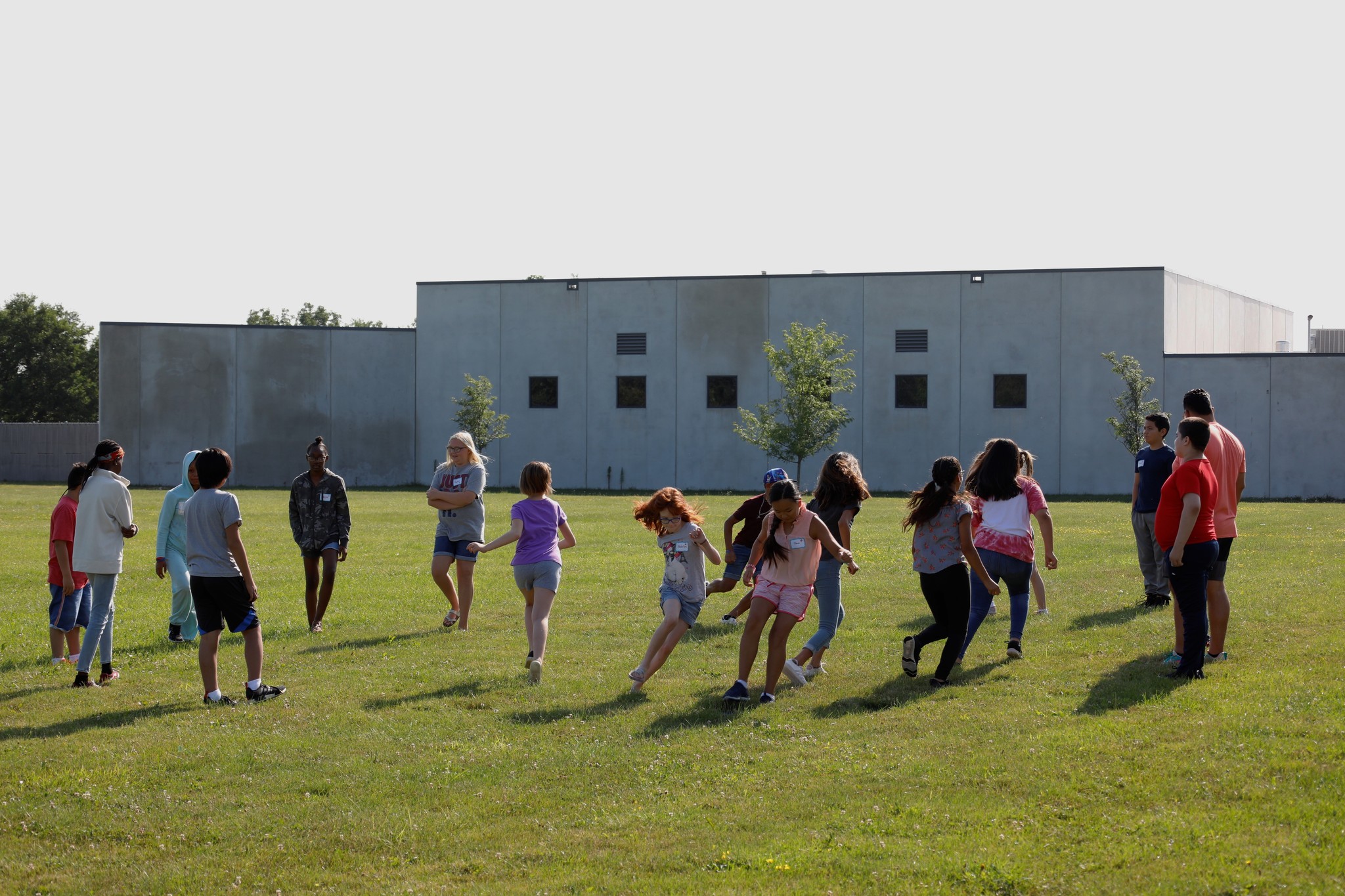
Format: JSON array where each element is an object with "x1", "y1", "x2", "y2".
[
  {"x1": 660, "y1": 515, "x2": 681, "y2": 524},
  {"x1": 444, "y1": 446, "x2": 466, "y2": 454}
]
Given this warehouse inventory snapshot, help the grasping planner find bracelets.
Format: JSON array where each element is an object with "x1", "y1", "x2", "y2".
[
  {"x1": 156, "y1": 558, "x2": 165, "y2": 562},
  {"x1": 698, "y1": 538, "x2": 707, "y2": 545},
  {"x1": 839, "y1": 557, "x2": 850, "y2": 565},
  {"x1": 725, "y1": 549, "x2": 734, "y2": 553},
  {"x1": 745, "y1": 564, "x2": 756, "y2": 572}
]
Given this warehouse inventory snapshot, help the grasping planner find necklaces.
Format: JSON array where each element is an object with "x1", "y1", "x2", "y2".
[{"x1": 756, "y1": 492, "x2": 773, "y2": 519}]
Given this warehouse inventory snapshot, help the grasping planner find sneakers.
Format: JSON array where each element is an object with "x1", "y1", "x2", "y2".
[
  {"x1": 168, "y1": 624, "x2": 184, "y2": 643},
  {"x1": 99, "y1": 668, "x2": 119, "y2": 684},
  {"x1": 71, "y1": 677, "x2": 102, "y2": 690},
  {"x1": 203, "y1": 692, "x2": 237, "y2": 707},
  {"x1": 245, "y1": 681, "x2": 287, "y2": 703},
  {"x1": 806, "y1": 663, "x2": 828, "y2": 676},
  {"x1": 782, "y1": 658, "x2": 807, "y2": 686},
  {"x1": 723, "y1": 680, "x2": 751, "y2": 701},
  {"x1": 760, "y1": 692, "x2": 773, "y2": 703}
]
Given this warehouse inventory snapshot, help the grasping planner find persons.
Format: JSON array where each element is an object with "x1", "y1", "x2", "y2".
[
  {"x1": 702, "y1": 468, "x2": 799, "y2": 625},
  {"x1": 782, "y1": 451, "x2": 872, "y2": 688},
  {"x1": 1131, "y1": 412, "x2": 1178, "y2": 609},
  {"x1": 954, "y1": 438, "x2": 1059, "y2": 666},
  {"x1": 156, "y1": 450, "x2": 200, "y2": 646},
  {"x1": 722, "y1": 477, "x2": 852, "y2": 706},
  {"x1": 1162, "y1": 388, "x2": 1245, "y2": 670},
  {"x1": 182, "y1": 446, "x2": 288, "y2": 708},
  {"x1": 48, "y1": 462, "x2": 92, "y2": 664},
  {"x1": 466, "y1": 461, "x2": 576, "y2": 686},
  {"x1": 1156, "y1": 417, "x2": 1219, "y2": 681},
  {"x1": 901, "y1": 456, "x2": 1002, "y2": 689},
  {"x1": 426, "y1": 431, "x2": 486, "y2": 631},
  {"x1": 289, "y1": 436, "x2": 351, "y2": 633},
  {"x1": 72, "y1": 438, "x2": 139, "y2": 688},
  {"x1": 622, "y1": 487, "x2": 721, "y2": 697}
]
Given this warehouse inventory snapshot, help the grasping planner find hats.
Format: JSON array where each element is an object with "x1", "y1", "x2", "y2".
[{"x1": 763, "y1": 468, "x2": 798, "y2": 484}]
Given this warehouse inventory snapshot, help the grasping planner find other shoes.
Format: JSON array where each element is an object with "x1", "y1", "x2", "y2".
[
  {"x1": 1138, "y1": 591, "x2": 1171, "y2": 607},
  {"x1": 1203, "y1": 652, "x2": 1227, "y2": 664},
  {"x1": 528, "y1": 659, "x2": 542, "y2": 688},
  {"x1": 929, "y1": 678, "x2": 955, "y2": 690},
  {"x1": 1161, "y1": 650, "x2": 1182, "y2": 665},
  {"x1": 721, "y1": 614, "x2": 739, "y2": 626},
  {"x1": 311, "y1": 621, "x2": 322, "y2": 633},
  {"x1": 1158, "y1": 666, "x2": 1205, "y2": 682},
  {"x1": 1005, "y1": 640, "x2": 1023, "y2": 660},
  {"x1": 987, "y1": 600, "x2": 997, "y2": 616},
  {"x1": 902, "y1": 635, "x2": 922, "y2": 677},
  {"x1": 525, "y1": 651, "x2": 533, "y2": 669}
]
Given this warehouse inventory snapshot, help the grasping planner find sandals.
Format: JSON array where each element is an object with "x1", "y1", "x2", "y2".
[
  {"x1": 443, "y1": 609, "x2": 460, "y2": 627},
  {"x1": 628, "y1": 668, "x2": 645, "y2": 682}
]
